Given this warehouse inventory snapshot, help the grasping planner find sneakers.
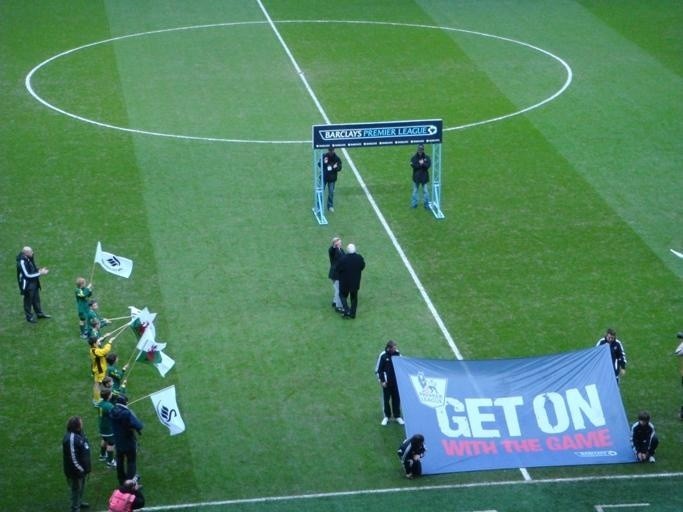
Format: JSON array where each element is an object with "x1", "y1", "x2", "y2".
[
  {"x1": 36, "y1": 313, "x2": 50, "y2": 318},
  {"x1": 92, "y1": 398, "x2": 103, "y2": 408},
  {"x1": 328, "y1": 206, "x2": 334, "y2": 213},
  {"x1": 71, "y1": 501, "x2": 89, "y2": 512},
  {"x1": 331, "y1": 301, "x2": 356, "y2": 319},
  {"x1": 649, "y1": 455, "x2": 656, "y2": 464},
  {"x1": 98, "y1": 451, "x2": 116, "y2": 469},
  {"x1": 78, "y1": 328, "x2": 88, "y2": 339},
  {"x1": 394, "y1": 416, "x2": 405, "y2": 425},
  {"x1": 24, "y1": 316, "x2": 37, "y2": 323},
  {"x1": 380, "y1": 416, "x2": 388, "y2": 426}
]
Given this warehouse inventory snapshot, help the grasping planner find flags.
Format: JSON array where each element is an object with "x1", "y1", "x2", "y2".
[
  {"x1": 94, "y1": 241, "x2": 133, "y2": 278},
  {"x1": 391, "y1": 355, "x2": 641, "y2": 475},
  {"x1": 127, "y1": 305, "x2": 176, "y2": 378},
  {"x1": 150, "y1": 385, "x2": 186, "y2": 436}
]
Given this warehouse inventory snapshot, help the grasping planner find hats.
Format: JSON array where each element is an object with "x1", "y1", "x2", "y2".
[
  {"x1": 417, "y1": 144, "x2": 424, "y2": 151},
  {"x1": 327, "y1": 147, "x2": 334, "y2": 151}
]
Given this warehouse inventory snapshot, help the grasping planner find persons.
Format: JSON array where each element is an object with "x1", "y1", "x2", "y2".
[
  {"x1": 74, "y1": 277, "x2": 144, "y2": 468},
  {"x1": 63, "y1": 416, "x2": 91, "y2": 512},
  {"x1": 108, "y1": 479, "x2": 145, "y2": 512},
  {"x1": 16, "y1": 246, "x2": 52, "y2": 324},
  {"x1": 397, "y1": 434, "x2": 426, "y2": 479},
  {"x1": 410, "y1": 144, "x2": 431, "y2": 209},
  {"x1": 328, "y1": 237, "x2": 346, "y2": 313},
  {"x1": 595, "y1": 329, "x2": 627, "y2": 384},
  {"x1": 317, "y1": 147, "x2": 342, "y2": 213},
  {"x1": 336, "y1": 244, "x2": 365, "y2": 318},
  {"x1": 108, "y1": 395, "x2": 144, "y2": 486},
  {"x1": 374, "y1": 340, "x2": 405, "y2": 426},
  {"x1": 629, "y1": 412, "x2": 659, "y2": 463}
]
